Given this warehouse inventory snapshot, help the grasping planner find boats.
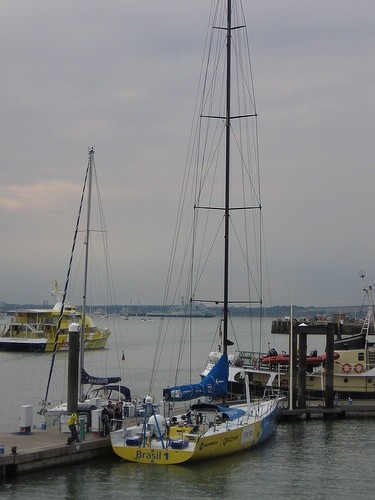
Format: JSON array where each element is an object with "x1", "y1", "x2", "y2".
[
  {"x1": 194, "y1": 269, "x2": 374, "y2": 398},
  {"x1": 0, "y1": 280, "x2": 112, "y2": 351}
]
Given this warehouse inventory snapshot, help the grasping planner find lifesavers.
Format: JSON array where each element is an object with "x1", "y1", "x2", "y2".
[
  {"x1": 354, "y1": 363, "x2": 363, "y2": 373},
  {"x1": 342, "y1": 363, "x2": 351, "y2": 373},
  {"x1": 280, "y1": 379, "x2": 288, "y2": 387}
]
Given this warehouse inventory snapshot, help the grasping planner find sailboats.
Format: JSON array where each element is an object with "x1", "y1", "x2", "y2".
[
  {"x1": 34, "y1": 144, "x2": 161, "y2": 435},
  {"x1": 108, "y1": 0, "x2": 292, "y2": 466}
]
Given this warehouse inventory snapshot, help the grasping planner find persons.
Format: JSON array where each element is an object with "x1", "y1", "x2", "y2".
[
  {"x1": 65, "y1": 410, "x2": 82, "y2": 446},
  {"x1": 100, "y1": 400, "x2": 124, "y2": 438},
  {"x1": 278, "y1": 314, "x2": 355, "y2": 326}
]
